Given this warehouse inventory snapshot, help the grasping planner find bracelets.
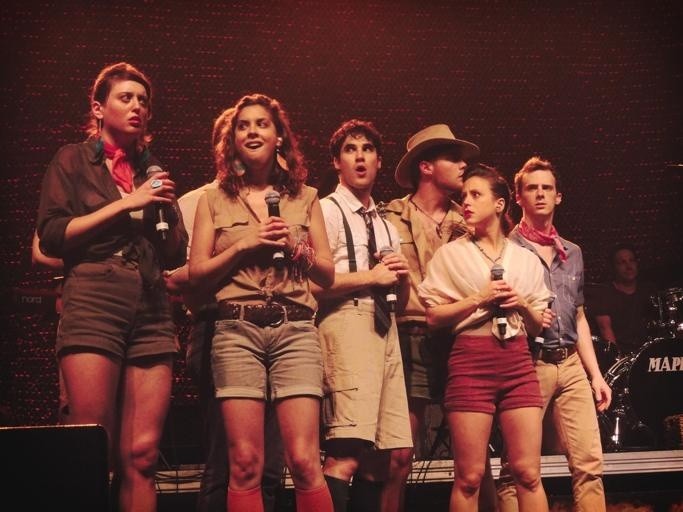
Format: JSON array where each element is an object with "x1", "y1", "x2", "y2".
[{"x1": 292, "y1": 242, "x2": 314, "y2": 272}]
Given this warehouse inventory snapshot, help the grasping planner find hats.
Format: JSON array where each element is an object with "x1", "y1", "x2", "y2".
[{"x1": 395, "y1": 124, "x2": 480, "y2": 188}]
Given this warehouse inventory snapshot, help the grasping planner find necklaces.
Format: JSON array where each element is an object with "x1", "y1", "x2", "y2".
[
  {"x1": 468, "y1": 232, "x2": 505, "y2": 263},
  {"x1": 409, "y1": 199, "x2": 450, "y2": 239}
]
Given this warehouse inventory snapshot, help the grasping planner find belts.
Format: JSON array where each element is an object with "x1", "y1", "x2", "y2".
[
  {"x1": 219, "y1": 303, "x2": 315, "y2": 327},
  {"x1": 528, "y1": 348, "x2": 579, "y2": 366}
]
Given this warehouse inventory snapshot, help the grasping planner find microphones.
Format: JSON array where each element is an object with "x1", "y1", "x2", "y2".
[
  {"x1": 265, "y1": 190, "x2": 285, "y2": 259},
  {"x1": 491, "y1": 264, "x2": 507, "y2": 336},
  {"x1": 379, "y1": 246, "x2": 397, "y2": 302},
  {"x1": 146, "y1": 164, "x2": 170, "y2": 242},
  {"x1": 532, "y1": 292, "x2": 556, "y2": 359}
]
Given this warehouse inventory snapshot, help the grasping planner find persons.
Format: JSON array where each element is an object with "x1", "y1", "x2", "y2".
[
  {"x1": 508, "y1": 155, "x2": 612, "y2": 512},
  {"x1": 595, "y1": 239, "x2": 659, "y2": 365},
  {"x1": 189, "y1": 94, "x2": 335, "y2": 512},
  {"x1": 41, "y1": 66, "x2": 190, "y2": 512},
  {"x1": 416, "y1": 164, "x2": 549, "y2": 512},
  {"x1": 31, "y1": 107, "x2": 285, "y2": 512},
  {"x1": 382, "y1": 123, "x2": 497, "y2": 512},
  {"x1": 306, "y1": 120, "x2": 411, "y2": 512}
]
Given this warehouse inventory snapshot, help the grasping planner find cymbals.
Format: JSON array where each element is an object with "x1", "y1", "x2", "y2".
[{"x1": 583, "y1": 281, "x2": 610, "y2": 296}]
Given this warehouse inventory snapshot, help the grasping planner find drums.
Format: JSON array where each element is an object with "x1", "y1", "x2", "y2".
[
  {"x1": 627, "y1": 337, "x2": 683, "y2": 432},
  {"x1": 657, "y1": 289, "x2": 683, "y2": 332}
]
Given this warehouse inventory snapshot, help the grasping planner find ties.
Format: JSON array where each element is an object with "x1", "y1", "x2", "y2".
[{"x1": 357, "y1": 207, "x2": 392, "y2": 338}]
